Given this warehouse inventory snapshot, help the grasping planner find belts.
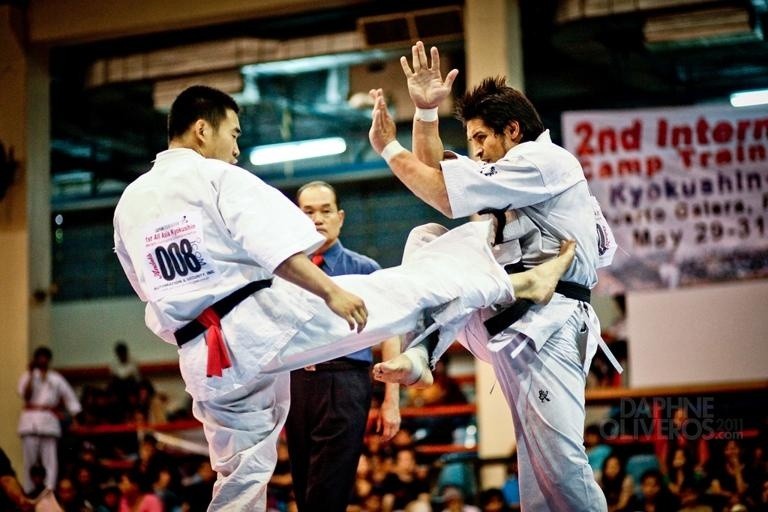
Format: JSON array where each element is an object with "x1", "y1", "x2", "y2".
[{"x1": 291, "y1": 361, "x2": 370, "y2": 373}]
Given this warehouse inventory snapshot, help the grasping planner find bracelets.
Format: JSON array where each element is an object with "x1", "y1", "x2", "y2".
[
  {"x1": 412, "y1": 106, "x2": 439, "y2": 124},
  {"x1": 381, "y1": 141, "x2": 404, "y2": 167}
]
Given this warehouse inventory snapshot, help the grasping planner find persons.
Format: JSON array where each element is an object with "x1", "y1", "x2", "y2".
[
  {"x1": 370, "y1": 40, "x2": 617, "y2": 512},
  {"x1": 0, "y1": 375, "x2": 766, "y2": 512},
  {"x1": 111, "y1": 341, "x2": 142, "y2": 383},
  {"x1": 15, "y1": 348, "x2": 84, "y2": 499},
  {"x1": 284, "y1": 180, "x2": 402, "y2": 512},
  {"x1": 115, "y1": 87, "x2": 577, "y2": 512}
]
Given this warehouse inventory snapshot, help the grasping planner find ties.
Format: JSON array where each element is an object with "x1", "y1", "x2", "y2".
[{"x1": 311, "y1": 254, "x2": 324, "y2": 267}]
